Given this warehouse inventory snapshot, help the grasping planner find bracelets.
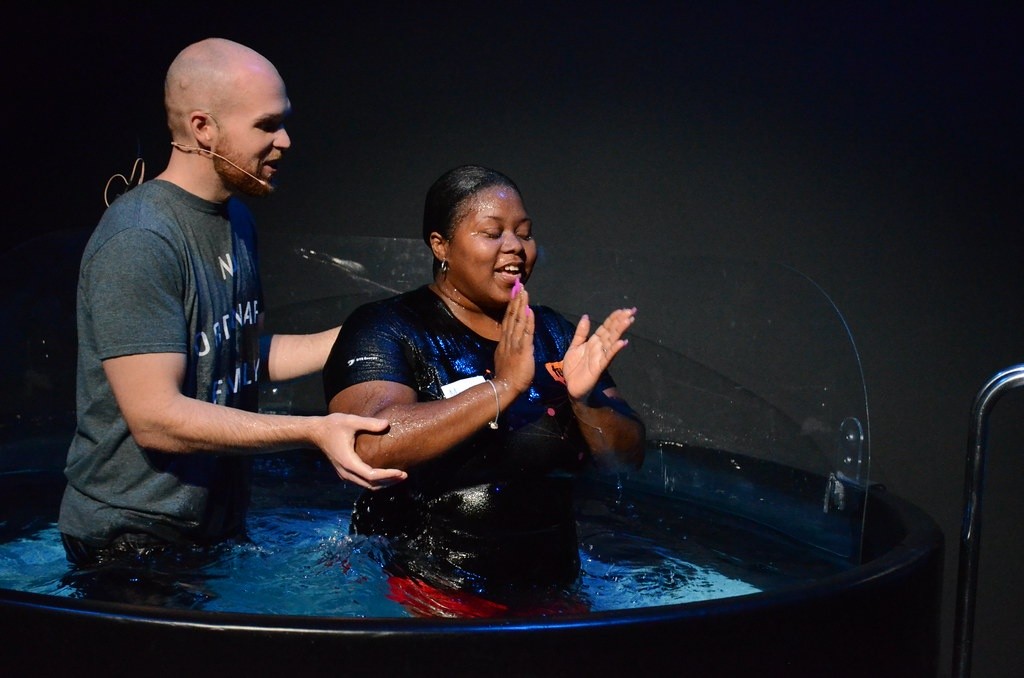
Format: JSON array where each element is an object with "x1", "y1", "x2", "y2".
[{"x1": 486, "y1": 379, "x2": 500, "y2": 430}]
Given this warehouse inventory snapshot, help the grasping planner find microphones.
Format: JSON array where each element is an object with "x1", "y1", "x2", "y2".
[{"x1": 172, "y1": 142, "x2": 274, "y2": 192}]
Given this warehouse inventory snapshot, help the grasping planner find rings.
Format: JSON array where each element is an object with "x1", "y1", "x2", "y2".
[{"x1": 602, "y1": 348, "x2": 606, "y2": 352}]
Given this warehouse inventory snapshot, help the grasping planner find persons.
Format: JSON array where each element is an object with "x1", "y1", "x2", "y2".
[
  {"x1": 59, "y1": 37, "x2": 408, "y2": 563},
  {"x1": 323, "y1": 163, "x2": 645, "y2": 576}
]
